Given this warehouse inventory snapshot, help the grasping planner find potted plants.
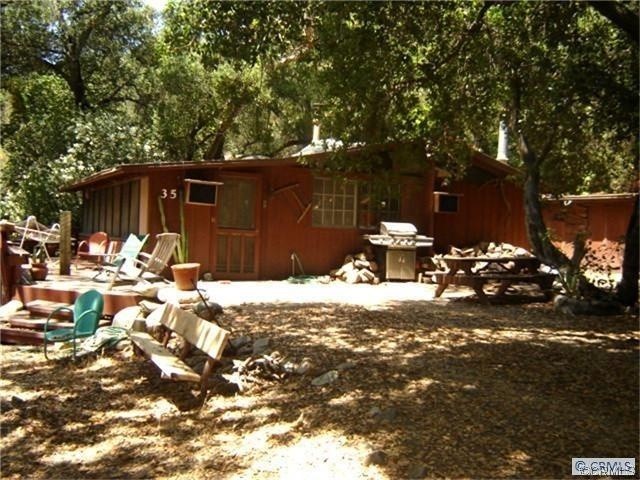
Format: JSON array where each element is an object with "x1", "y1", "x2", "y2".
[{"x1": 28, "y1": 243, "x2": 48, "y2": 280}]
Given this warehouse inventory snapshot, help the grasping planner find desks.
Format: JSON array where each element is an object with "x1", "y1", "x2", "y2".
[{"x1": 432, "y1": 254, "x2": 545, "y2": 309}]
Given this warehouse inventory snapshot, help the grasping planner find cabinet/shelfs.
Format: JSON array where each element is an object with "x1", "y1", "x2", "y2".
[{"x1": 371, "y1": 248, "x2": 417, "y2": 282}]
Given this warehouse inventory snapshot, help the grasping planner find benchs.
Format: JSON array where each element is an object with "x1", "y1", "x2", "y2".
[
  {"x1": 124, "y1": 300, "x2": 231, "y2": 407},
  {"x1": 422, "y1": 267, "x2": 559, "y2": 307}
]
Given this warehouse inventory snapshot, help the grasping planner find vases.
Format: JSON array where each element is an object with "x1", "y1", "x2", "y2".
[{"x1": 170, "y1": 262, "x2": 200, "y2": 291}]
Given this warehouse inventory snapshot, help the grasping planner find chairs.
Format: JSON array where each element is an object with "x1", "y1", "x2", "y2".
[
  {"x1": 40, "y1": 286, "x2": 104, "y2": 364},
  {"x1": 75, "y1": 230, "x2": 181, "y2": 290}
]
